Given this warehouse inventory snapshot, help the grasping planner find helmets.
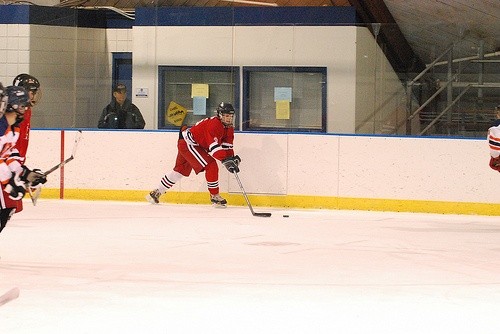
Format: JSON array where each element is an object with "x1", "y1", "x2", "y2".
[
  {"x1": 216, "y1": 101, "x2": 235, "y2": 114},
  {"x1": 2, "y1": 86, "x2": 32, "y2": 113},
  {"x1": 13, "y1": 74, "x2": 40, "y2": 92}
]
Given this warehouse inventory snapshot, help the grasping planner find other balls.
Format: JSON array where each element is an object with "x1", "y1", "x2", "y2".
[{"x1": 283, "y1": 215, "x2": 290, "y2": 217}]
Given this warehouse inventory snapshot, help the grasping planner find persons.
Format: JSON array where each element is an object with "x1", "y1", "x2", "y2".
[
  {"x1": 150, "y1": 102, "x2": 240, "y2": 205},
  {"x1": 487, "y1": 105, "x2": 500, "y2": 173},
  {"x1": 98, "y1": 83, "x2": 146, "y2": 129},
  {"x1": 0, "y1": 74, "x2": 47, "y2": 234}
]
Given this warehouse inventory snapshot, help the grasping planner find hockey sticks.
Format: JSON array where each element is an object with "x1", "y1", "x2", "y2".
[
  {"x1": 25, "y1": 129, "x2": 82, "y2": 188},
  {"x1": 233, "y1": 169, "x2": 272, "y2": 218}
]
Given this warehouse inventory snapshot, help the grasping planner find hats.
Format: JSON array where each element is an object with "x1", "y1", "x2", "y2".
[{"x1": 114, "y1": 84, "x2": 128, "y2": 93}]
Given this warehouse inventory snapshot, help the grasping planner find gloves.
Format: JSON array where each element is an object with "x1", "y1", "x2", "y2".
[
  {"x1": 489, "y1": 154, "x2": 500, "y2": 173},
  {"x1": 222, "y1": 157, "x2": 240, "y2": 173},
  {"x1": 2, "y1": 171, "x2": 26, "y2": 201},
  {"x1": 22, "y1": 168, "x2": 47, "y2": 186},
  {"x1": 231, "y1": 155, "x2": 241, "y2": 166}
]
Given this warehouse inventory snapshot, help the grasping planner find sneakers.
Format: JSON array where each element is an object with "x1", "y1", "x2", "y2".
[
  {"x1": 146, "y1": 187, "x2": 161, "y2": 204},
  {"x1": 210, "y1": 194, "x2": 227, "y2": 207}
]
